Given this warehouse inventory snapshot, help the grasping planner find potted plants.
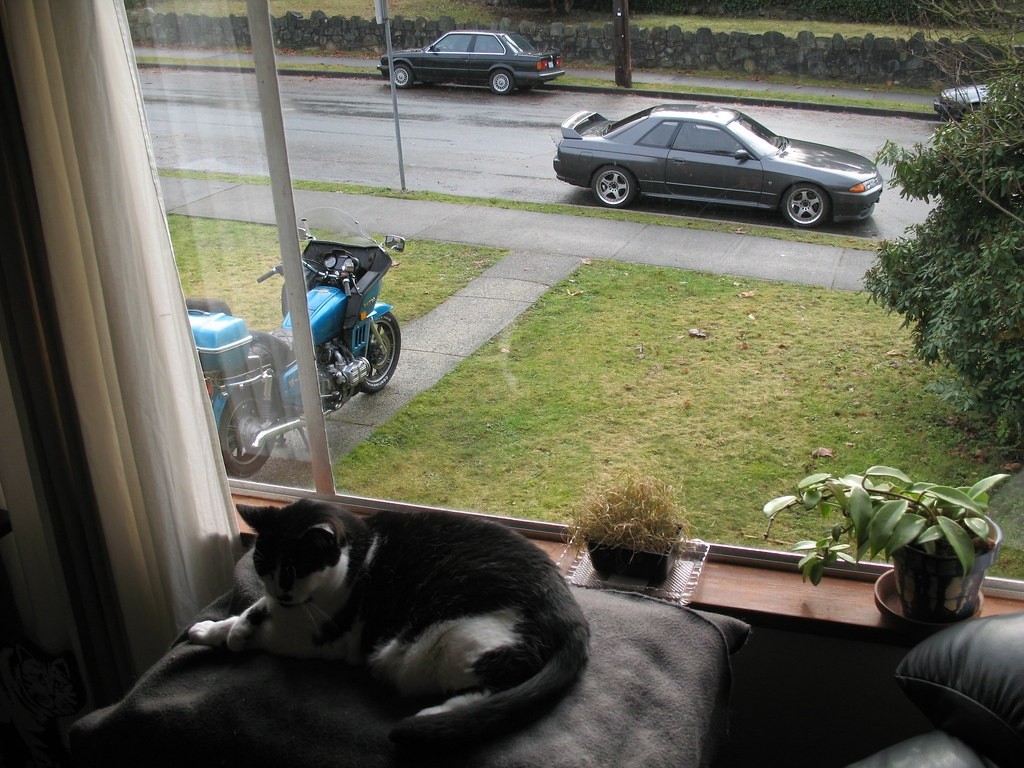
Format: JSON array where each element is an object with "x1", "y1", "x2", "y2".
[
  {"x1": 560, "y1": 470, "x2": 716, "y2": 583},
  {"x1": 762, "y1": 466, "x2": 1010, "y2": 623}
]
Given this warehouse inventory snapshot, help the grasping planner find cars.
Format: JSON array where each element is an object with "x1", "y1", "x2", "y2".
[
  {"x1": 377, "y1": 30, "x2": 565, "y2": 95},
  {"x1": 933, "y1": 82, "x2": 1018, "y2": 125},
  {"x1": 554, "y1": 104, "x2": 883, "y2": 229}
]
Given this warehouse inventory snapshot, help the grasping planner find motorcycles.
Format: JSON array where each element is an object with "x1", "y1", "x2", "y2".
[{"x1": 184, "y1": 207, "x2": 406, "y2": 478}]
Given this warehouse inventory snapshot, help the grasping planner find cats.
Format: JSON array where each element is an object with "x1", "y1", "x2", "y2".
[{"x1": 188, "y1": 500, "x2": 591, "y2": 754}]
